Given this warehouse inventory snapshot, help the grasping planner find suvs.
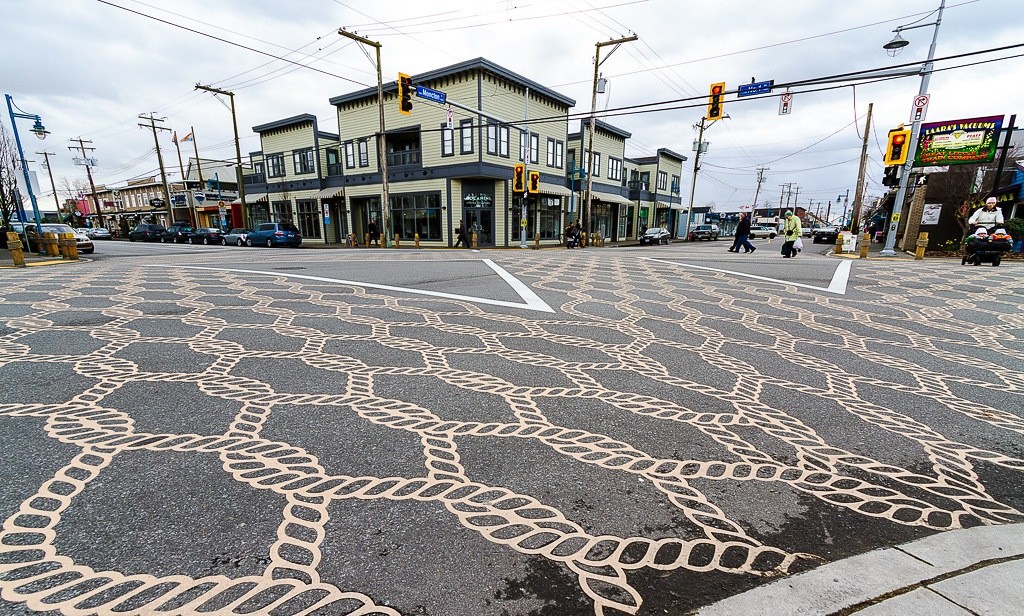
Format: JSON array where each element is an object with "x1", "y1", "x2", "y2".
[
  {"x1": 161, "y1": 222, "x2": 195, "y2": 244},
  {"x1": 692, "y1": 224, "x2": 720, "y2": 241},
  {"x1": 128, "y1": 223, "x2": 165, "y2": 242},
  {"x1": 749, "y1": 226, "x2": 778, "y2": 239},
  {"x1": 245, "y1": 222, "x2": 302, "y2": 248}
]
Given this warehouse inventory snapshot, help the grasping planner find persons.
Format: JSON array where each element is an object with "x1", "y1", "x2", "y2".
[
  {"x1": 783, "y1": 211, "x2": 802, "y2": 258},
  {"x1": 727, "y1": 213, "x2": 756, "y2": 254},
  {"x1": 965, "y1": 197, "x2": 1013, "y2": 265},
  {"x1": 368, "y1": 219, "x2": 379, "y2": 247},
  {"x1": 870, "y1": 224, "x2": 877, "y2": 243},
  {"x1": 454, "y1": 220, "x2": 470, "y2": 248},
  {"x1": 642, "y1": 224, "x2": 647, "y2": 233},
  {"x1": 566, "y1": 219, "x2": 584, "y2": 248}
]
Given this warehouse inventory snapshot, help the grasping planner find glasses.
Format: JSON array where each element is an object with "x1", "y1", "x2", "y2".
[{"x1": 786, "y1": 216, "x2": 789, "y2": 219}]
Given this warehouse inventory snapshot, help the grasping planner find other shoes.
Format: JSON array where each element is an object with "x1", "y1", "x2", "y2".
[
  {"x1": 727, "y1": 249, "x2": 733, "y2": 252},
  {"x1": 792, "y1": 250, "x2": 797, "y2": 257},
  {"x1": 783, "y1": 254, "x2": 790, "y2": 258},
  {"x1": 743, "y1": 250, "x2": 750, "y2": 253},
  {"x1": 733, "y1": 251, "x2": 739, "y2": 253},
  {"x1": 750, "y1": 247, "x2": 756, "y2": 254}
]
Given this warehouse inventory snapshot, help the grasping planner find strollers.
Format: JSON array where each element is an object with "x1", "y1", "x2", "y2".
[{"x1": 961, "y1": 221, "x2": 1013, "y2": 266}]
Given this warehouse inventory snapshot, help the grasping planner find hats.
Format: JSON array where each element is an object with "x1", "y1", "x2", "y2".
[
  {"x1": 975, "y1": 228, "x2": 987, "y2": 235},
  {"x1": 986, "y1": 197, "x2": 996, "y2": 205},
  {"x1": 995, "y1": 228, "x2": 1006, "y2": 235},
  {"x1": 785, "y1": 211, "x2": 792, "y2": 216}
]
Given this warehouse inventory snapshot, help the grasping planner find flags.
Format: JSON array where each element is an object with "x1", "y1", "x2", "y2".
[{"x1": 181, "y1": 133, "x2": 194, "y2": 142}]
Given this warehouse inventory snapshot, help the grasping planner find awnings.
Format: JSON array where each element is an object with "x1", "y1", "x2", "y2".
[
  {"x1": 231, "y1": 194, "x2": 267, "y2": 204},
  {"x1": 585, "y1": 191, "x2": 634, "y2": 205},
  {"x1": 526, "y1": 182, "x2": 581, "y2": 197},
  {"x1": 657, "y1": 202, "x2": 688, "y2": 210},
  {"x1": 311, "y1": 187, "x2": 344, "y2": 199}
]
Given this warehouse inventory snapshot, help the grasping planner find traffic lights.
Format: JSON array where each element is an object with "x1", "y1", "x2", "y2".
[
  {"x1": 915, "y1": 174, "x2": 928, "y2": 185},
  {"x1": 529, "y1": 171, "x2": 540, "y2": 193},
  {"x1": 708, "y1": 81, "x2": 726, "y2": 121},
  {"x1": 513, "y1": 163, "x2": 525, "y2": 192},
  {"x1": 882, "y1": 167, "x2": 893, "y2": 186},
  {"x1": 884, "y1": 130, "x2": 912, "y2": 164},
  {"x1": 398, "y1": 72, "x2": 413, "y2": 115}
]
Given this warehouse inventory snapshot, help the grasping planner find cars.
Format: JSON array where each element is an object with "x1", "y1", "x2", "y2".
[
  {"x1": 78, "y1": 228, "x2": 113, "y2": 240},
  {"x1": 801, "y1": 222, "x2": 843, "y2": 245},
  {"x1": 188, "y1": 227, "x2": 226, "y2": 245},
  {"x1": 638, "y1": 227, "x2": 671, "y2": 245},
  {"x1": 8, "y1": 222, "x2": 95, "y2": 254},
  {"x1": 222, "y1": 228, "x2": 253, "y2": 247}
]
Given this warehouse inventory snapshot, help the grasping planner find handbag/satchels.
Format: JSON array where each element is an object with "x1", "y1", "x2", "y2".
[
  {"x1": 793, "y1": 237, "x2": 803, "y2": 249},
  {"x1": 787, "y1": 230, "x2": 794, "y2": 236}
]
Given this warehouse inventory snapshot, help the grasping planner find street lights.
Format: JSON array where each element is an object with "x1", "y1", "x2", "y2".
[
  {"x1": 570, "y1": 160, "x2": 587, "y2": 222},
  {"x1": 4, "y1": 93, "x2": 52, "y2": 254},
  {"x1": 837, "y1": 189, "x2": 849, "y2": 229},
  {"x1": 668, "y1": 186, "x2": 681, "y2": 232},
  {"x1": 879, "y1": 0, "x2": 947, "y2": 258}
]
